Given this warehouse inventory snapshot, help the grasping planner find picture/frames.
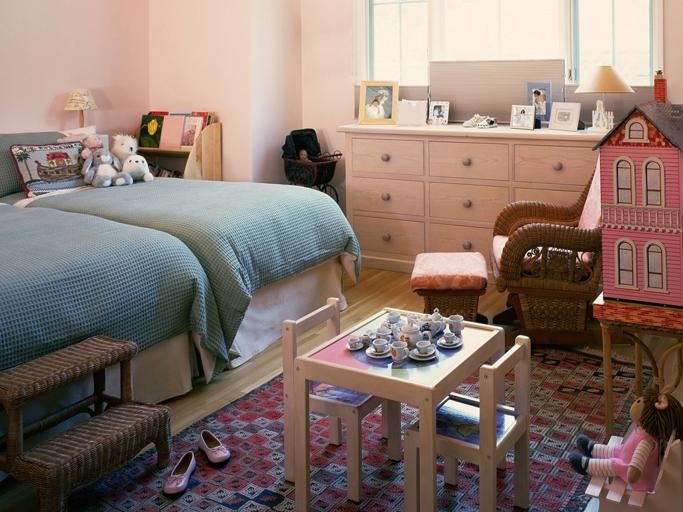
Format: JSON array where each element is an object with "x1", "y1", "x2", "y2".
[
  {"x1": 548, "y1": 101, "x2": 580, "y2": 131},
  {"x1": 510, "y1": 105, "x2": 535, "y2": 130},
  {"x1": 359, "y1": 80, "x2": 399, "y2": 126},
  {"x1": 427, "y1": 100, "x2": 450, "y2": 126},
  {"x1": 527, "y1": 81, "x2": 551, "y2": 121}
]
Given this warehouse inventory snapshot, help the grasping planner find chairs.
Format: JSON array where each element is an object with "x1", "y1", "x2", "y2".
[
  {"x1": 488, "y1": 150, "x2": 626, "y2": 345},
  {"x1": 402, "y1": 335, "x2": 529, "y2": 512},
  {"x1": 280, "y1": 296, "x2": 402, "y2": 505},
  {"x1": 584, "y1": 428, "x2": 676, "y2": 508}
]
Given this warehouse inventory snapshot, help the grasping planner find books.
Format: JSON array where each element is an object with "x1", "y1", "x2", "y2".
[{"x1": 138, "y1": 111, "x2": 215, "y2": 178}]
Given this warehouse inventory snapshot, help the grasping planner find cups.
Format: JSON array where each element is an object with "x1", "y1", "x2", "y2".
[{"x1": 346, "y1": 309, "x2": 464, "y2": 362}]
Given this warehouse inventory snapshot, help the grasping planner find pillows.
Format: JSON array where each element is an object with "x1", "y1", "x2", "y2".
[
  {"x1": 0, "y1": 131, "x2": 68, "y2": 197},
  {"x1": 10, "y1": 142, "x2": 94, "y2": 198},
  {"x1": 59, "y1": 126, "x2": 97, "y2": 137}
]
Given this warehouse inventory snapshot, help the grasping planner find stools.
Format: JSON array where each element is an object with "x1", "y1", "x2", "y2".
[
  {"x1": 410, "y1": 252, "x2": 489, "y2": 322},
  {"x1": 600, "y1": 320, "x2": 682, "y2": 440}
]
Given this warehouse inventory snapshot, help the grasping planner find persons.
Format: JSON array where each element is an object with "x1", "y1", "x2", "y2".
[
  {"x1": 532, "y1": 89, "x2": 546, "y2": 116},
  {"x1": 433, "y1": 106, "x2": 444, "y2": 119},
  {"x1": 368, "y1": 90, "x2": 387, "y2": 115},
  {"x1": 365, "y1": 98, "x2": 380, "y2": 117}
]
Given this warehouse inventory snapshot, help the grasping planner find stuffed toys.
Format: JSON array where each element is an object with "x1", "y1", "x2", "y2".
[
  {"x1": 79, "y1": 147, "x2": 132, "y2": 187},
  {"x1": 565, "y1": 380, "x2": 682, "y2": 493},
  {"x1": 120, "y1": 154, "x2": 153, "y2": 182},
  {"x1": 108, "y1": 131, "x2": 137, "y2": 162},
  {"x1": 79, "y1": 135, "x2": 104, "y2": 160}
]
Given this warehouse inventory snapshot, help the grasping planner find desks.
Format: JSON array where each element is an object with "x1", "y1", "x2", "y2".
[{"x1": 293, "y1": 306, "x2": 507, "y2": 511}]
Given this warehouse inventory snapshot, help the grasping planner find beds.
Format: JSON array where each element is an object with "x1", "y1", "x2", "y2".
[
  {"x1": 0, "y1": 202, "x2": 229, "y2": 481},
  {"x1": 1, "y1": 176, "x2": 362, "y2": 371}
]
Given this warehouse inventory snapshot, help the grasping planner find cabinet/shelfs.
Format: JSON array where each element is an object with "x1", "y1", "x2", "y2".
[
  {"x1": 130, "y1": 121, "x2": 222, "y2": 181},
  {"x1": 336, "y1": 124, "x2": 608, "y2": 287}
]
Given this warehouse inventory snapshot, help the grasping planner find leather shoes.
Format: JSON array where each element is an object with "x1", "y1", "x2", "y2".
[
  {"x1": 162, "y1": 450, "x2": 196, "y2": 494},
  {"x1": 198, "y1": 430, "x2": 231, "y2": 465}
]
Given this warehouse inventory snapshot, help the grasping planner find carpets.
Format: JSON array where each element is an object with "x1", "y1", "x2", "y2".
[{"x1": 0, "y1": 344, "x2": 657, "y2": 511}]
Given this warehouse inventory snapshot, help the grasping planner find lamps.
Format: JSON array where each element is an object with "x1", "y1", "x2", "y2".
[
  {"x1": 64, "y1": 89, "x2": 97, "y2": 128},
  {"x1": 573, "y1": 65, "x2": 637, "y2": 132}
]
